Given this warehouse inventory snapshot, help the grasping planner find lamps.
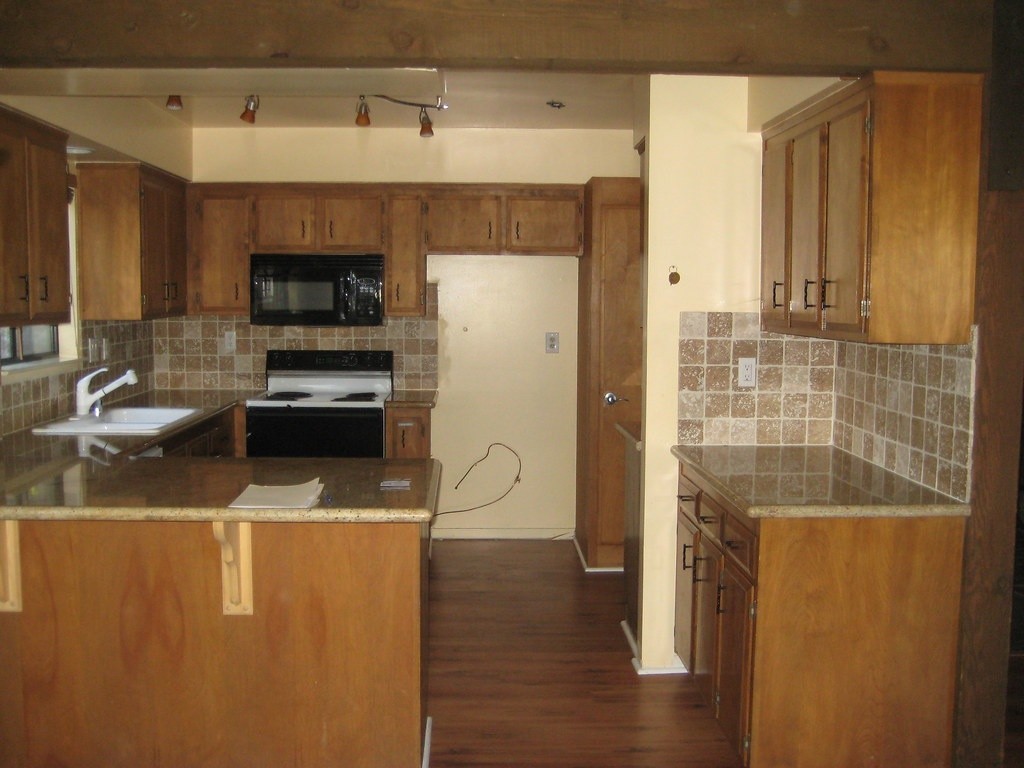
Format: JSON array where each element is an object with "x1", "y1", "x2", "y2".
[
  {"x1": 240, "y1": 93, "x2": 260, "y2": 124},
  {"x1": 356, "y1": 91, "x2": 447, "y2": 138},
  {"x1": 165, "y1": 93, "x2": 183, "y2": 109}
]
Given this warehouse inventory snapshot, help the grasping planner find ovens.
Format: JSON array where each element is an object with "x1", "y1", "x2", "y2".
[{"x1": 245, "y1": 406, "x2": 386, "y2": 459}]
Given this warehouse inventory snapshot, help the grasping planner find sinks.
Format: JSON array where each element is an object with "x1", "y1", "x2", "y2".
[{"x1": 48, "y1": 408, "x2": 198, "y2": 431}]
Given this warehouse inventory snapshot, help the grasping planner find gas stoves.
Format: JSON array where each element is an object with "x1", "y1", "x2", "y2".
[{"x1": 246, "y1": 350, "x2": 393, "y2": 407}]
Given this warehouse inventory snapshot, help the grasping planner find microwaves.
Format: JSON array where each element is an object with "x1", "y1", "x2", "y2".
[{"x1": 250, "y1": 253, "x2": 385, "y2": 326}]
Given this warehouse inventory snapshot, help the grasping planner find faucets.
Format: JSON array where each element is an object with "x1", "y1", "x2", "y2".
[{"x1": 74, "y1": 367, "x2": 138, "y2": 412}]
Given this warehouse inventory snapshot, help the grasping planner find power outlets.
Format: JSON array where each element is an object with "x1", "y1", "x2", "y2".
[
  {"x1": 102, "y1": 338, "x2": 112, "y2": 360},
  {"x1": 547, "y1": 332, "x2": 560, "y2": 353},
  {"x1": 738, "y1": 356, "x2": 756, "y2": 386},
  {"x1": 225, "y1": 332, "x2": 235, "y2": 351}
]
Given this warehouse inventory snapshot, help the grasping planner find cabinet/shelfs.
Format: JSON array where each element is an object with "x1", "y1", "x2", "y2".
[
  {"x1": 80, "y1": 159, "x2": 183, "y2": 322},
  {"x1": 757, "y1": 68, "x2": 994, "y2": 343},
  {"x1": 251, "y1": 182, "x2": 383, "y2": 252},
  {"x1": 574, "y1": 176, "x2": 649, "y2": 571},
  {"x1": 184, "y1": 181, "x2": 249, "y2": 316},
  {"x1": 423, "y1": 183, "x2": 587, "y2": 256},
  {"x1": 0, "y1": 103, "x2": 72, "y2": 324},
  {"x1": 152, "y1": 419, "x2": 237, "y2": 459},
  {"x1": 385, "y1": 408, "x2": 430, "y2": 462},
  {"x1": 673, "y1": 473, "x2": 764, "y2": 767},
  {"x1": 384, "y1": 183, "x2": 421, "y2": 314}
]
